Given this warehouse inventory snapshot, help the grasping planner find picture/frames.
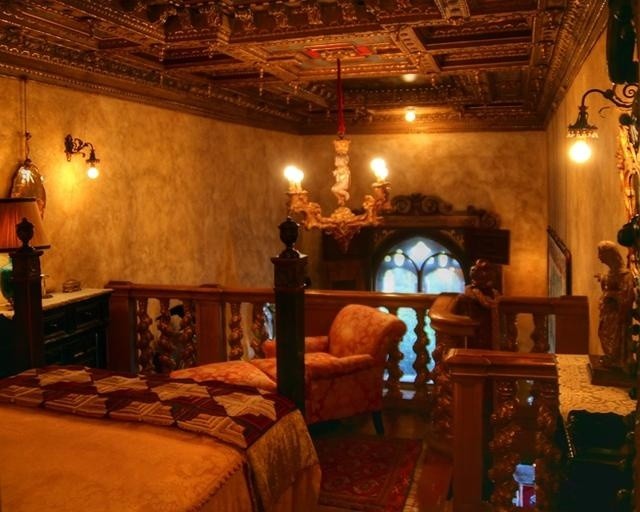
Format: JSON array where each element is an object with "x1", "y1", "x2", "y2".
[{"x1": 545, "y1": 224, "x2": 572, "y2": 354}]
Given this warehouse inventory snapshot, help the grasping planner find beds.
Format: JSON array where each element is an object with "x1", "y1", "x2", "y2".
[{"x1": 0, "y1": 216, "x2": 324, "y2": 512}]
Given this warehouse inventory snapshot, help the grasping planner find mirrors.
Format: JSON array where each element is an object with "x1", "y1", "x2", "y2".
[{"x1": 9, "y1": 75, "x2": 46, "y2": 220}]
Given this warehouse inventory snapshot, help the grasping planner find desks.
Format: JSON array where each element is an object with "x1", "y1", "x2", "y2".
[{"x1": 552, "y1": 352, "x2": 638, "y2": 467}]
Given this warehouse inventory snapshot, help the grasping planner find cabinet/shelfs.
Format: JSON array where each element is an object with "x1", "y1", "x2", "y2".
[{"x1": 0, "y1": 293, "x2": 112, "y2": 377}]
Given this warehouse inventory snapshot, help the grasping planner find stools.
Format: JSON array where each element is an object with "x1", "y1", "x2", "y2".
[{"x1": 167, "y1": 358, "x2": 277, "y2": 390}]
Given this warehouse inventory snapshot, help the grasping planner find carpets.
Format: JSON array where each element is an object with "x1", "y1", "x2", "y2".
[{"x1": 305, "y1": 429, "x2": 422, "y2": 512}]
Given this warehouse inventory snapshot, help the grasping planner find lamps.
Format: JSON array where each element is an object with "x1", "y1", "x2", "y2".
[
  {"x1": 0, "y1": 198, "x2": 51, "y2": 310},
  {"x1": 64, "y1": 134, "x2": 100, "y2": 179},
  {"x1": 566, "y1": 81, "x2": 640, "y2": 163},
  {"x1": 282, "y1": 59, "x2": 393, "y2": 247}
]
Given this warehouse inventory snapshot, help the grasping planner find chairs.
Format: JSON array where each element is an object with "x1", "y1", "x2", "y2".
[{"x1": 243, "y1": 305, "x2": 407, "y2": 437}]
